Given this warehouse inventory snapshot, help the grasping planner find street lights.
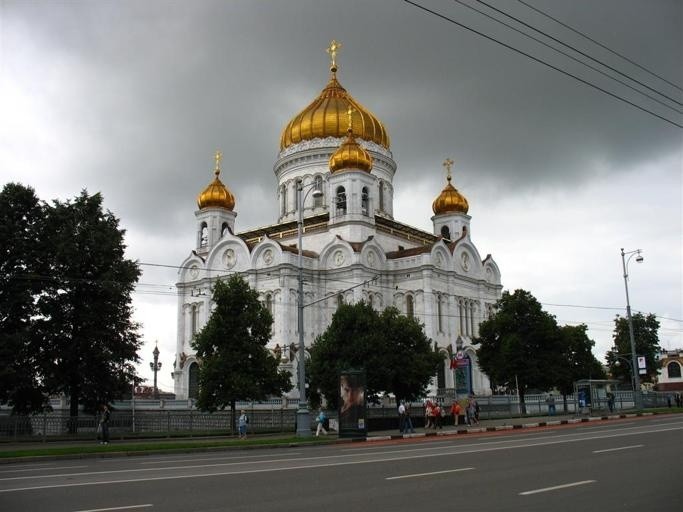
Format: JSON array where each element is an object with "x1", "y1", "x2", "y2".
[
  {"x1": 150, "y1": 340, "x2": 162, "y2": 398},
  {"x1": 620, "y1": 248, "x2": 644, "y2": 413},
  {"x1": 295, "y1": 179, "x2": 322, "y2": 438}
]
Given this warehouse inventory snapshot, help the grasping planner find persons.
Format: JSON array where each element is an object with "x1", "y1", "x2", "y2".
[
  {"x1": 339, "y1": 374, "x2": 365, "y2": 432},
  {"x1": 237, "y1": 409, "x2": 248, "y2": 440},
  {"x1": 666, "y1": 392, "x2": 682, "y2": 408},
  {"x1": 315, "y1": 407, "x2": 328, "y2": 436},
  {"x1": 545, "y1": 393, "x2": 555, "y2": 416},
  {"x1": 606, "y1": 392, "x2": 614, "y2": 414},
  {"x1": 99, "y1": 404, "x2": 112, "y2": 445},
  {"x1": 397, "y1": 393, "x2": 480, "y2": 434}
]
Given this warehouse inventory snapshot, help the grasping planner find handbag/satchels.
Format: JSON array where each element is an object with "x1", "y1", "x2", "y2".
[
  {"x1": 245, "y1": 417, "x2": 249, "y2": 423},
  {"x1": 316, "y1": 417, "x2": 320, "y2": 422}
]
